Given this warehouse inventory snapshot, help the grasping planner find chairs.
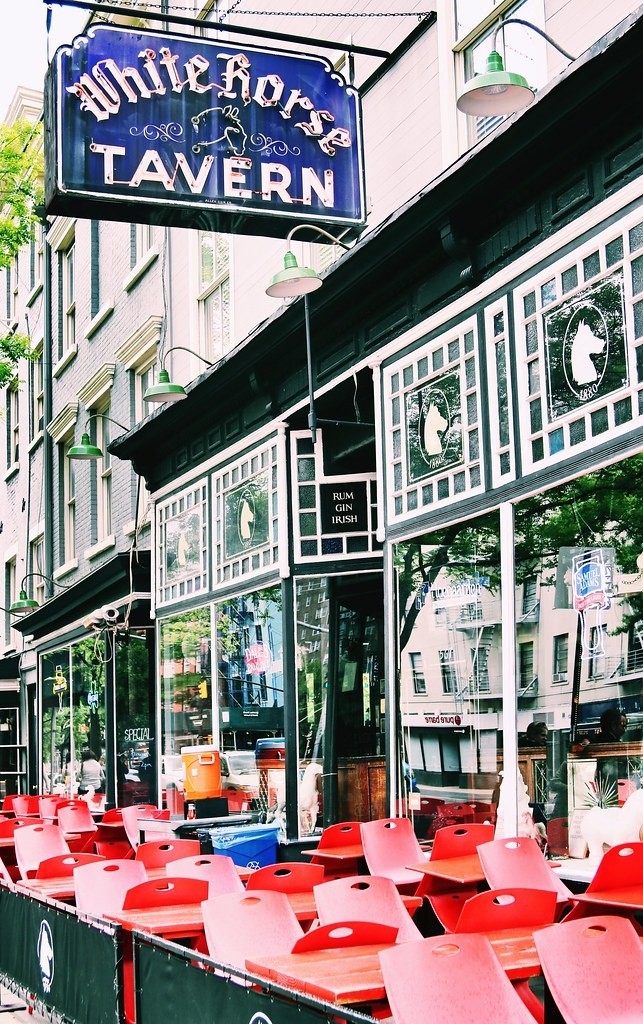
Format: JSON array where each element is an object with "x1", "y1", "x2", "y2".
[{"x1": 0, "y1": 793, "x2": 643, "y2": 1024}]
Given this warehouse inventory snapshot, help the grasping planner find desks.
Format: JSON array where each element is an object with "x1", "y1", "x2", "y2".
[
  {"x1": 18, "y1": 864, "x2": 257, "y2": 900},
  {"x1": 102, "y1": 889, "x2": 424, "y2": 973},
  {"x1": 403, "y1": 853, "x2": 561, "y2": 902},
  {"x1": 567, "y1": 884, "x2": 643, "y2": 928},
  {"x1": 137, "y1": 814, "x2": 252, "y2": 844},
  {"x1": 0, "y1": 809, "x2": 14, "y2": 814},
  {"x1": 94, "y1": 821, "x2": 125, "y2": 828},
  {"x1": 244, "y1": 921, "x2": 643, "y2": 1024},
  {"x1": 300, "y1": 843, "x2": 431, "y2": 889},
  {"x1": 17, "y1": 812, "x2": 40, "y2": 817},
  {"x1": 0, "y1": 833, "x2": 81, "y2": 848}
]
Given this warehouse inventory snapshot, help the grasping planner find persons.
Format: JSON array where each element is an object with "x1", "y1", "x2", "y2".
[
  {"x1": 78, "y1": 750, "x2": 105, "y2": 795},
  {"x1": 518, "y1": 722, "x2": 548, "y2": 748},
  {"x1": 117, "y1": 753, "x2": 130, "y2": 807},
  {"x1": 589, "y1": 709, "x2": 627, "y2": 744},
  {"x1": 551, "y1": 735, "x2": 590, "y2": 818}
]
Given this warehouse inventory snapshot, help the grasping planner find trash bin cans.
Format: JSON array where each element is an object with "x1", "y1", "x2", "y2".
[
  {"x1": 183, "y1": 797, "x2": 229, "y2": 820},
  {"x1": 172, "y1": 814, "x2": 259, "y2": 862},
  {"x1": 196, "y1": 822, "x2": 282, "y2": 888}
]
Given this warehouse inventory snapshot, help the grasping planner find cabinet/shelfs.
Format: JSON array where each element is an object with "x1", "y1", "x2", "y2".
[{"x1": 265, "y1": 740, "x2": 643, "y2": 826}]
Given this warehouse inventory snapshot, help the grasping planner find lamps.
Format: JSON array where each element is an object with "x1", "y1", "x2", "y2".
[
  {"x1": 265, "y1": 224, "x2": 351, "y2": 298},
  {"x1": 143, "y1": 347, "x2": 212, "y2": 402},
  {"x1": 66, "y1": 414, "x2": 130, "y2": 460},
  {"x1": 455, "y1": 19, "x2": 576, "y2": 117},
  {"x1": 8, "y1": 573, "x2": 69, "y2": 613}
]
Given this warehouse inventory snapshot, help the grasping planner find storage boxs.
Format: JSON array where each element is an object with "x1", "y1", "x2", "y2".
[
  {"x1": 257, "y1": 743, "x2": 284, "y2": 770},
  {"x1": 181, "y1": 746, "x2": 222, "y2": 801}
]
